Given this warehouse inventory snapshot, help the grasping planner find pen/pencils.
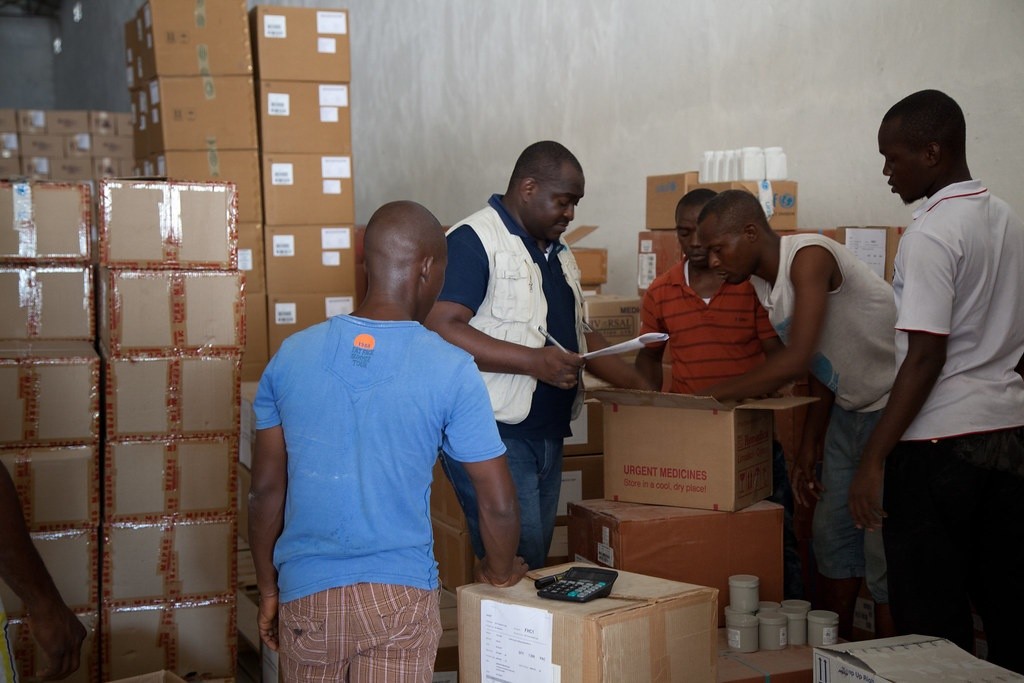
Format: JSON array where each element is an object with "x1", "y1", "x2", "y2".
[{"x1": 538, "y1": 325, "x2": 569, "y2": 353}]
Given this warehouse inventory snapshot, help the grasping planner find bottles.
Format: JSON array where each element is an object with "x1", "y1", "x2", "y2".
[
  {"x1": 725, "y1": 574, "x2": 839, "y2": 652},
  {"x1": 698, "y1": 146, "x2": 789, "y2": 183}
]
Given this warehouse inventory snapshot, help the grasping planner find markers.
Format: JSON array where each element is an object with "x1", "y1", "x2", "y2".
[{"x1": 534, "y1": 572, "x2": 565, "y2": 590}]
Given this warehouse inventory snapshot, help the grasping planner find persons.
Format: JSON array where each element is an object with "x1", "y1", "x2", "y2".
[
  {"x1": 850, "y1": 90, "x2": 1024, "y2": 676},
  {"x1": 635, "y1": 187, "x2": 802, "y2": 601},
  {"x1": 0, "y1": 461, "x2": 88, "y2": 683},
  {"x1": 697, "y1": 189, "x2": 896, "y2": 640},
  {"x1": 423, "y1": 140, "x2": 659, "y2": 568},
  {"x1": 248, "y1": 201, "x2": 529, "y2": 683}
]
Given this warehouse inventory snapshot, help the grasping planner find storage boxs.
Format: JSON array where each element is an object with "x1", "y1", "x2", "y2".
[{"x1": 0, "y1": 0, "x2": 1024, "y2": 683}]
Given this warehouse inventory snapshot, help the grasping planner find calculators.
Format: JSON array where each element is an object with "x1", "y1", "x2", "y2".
[{"x1": 536, "y1": 567, "x2": 618, "y2": 603}]
